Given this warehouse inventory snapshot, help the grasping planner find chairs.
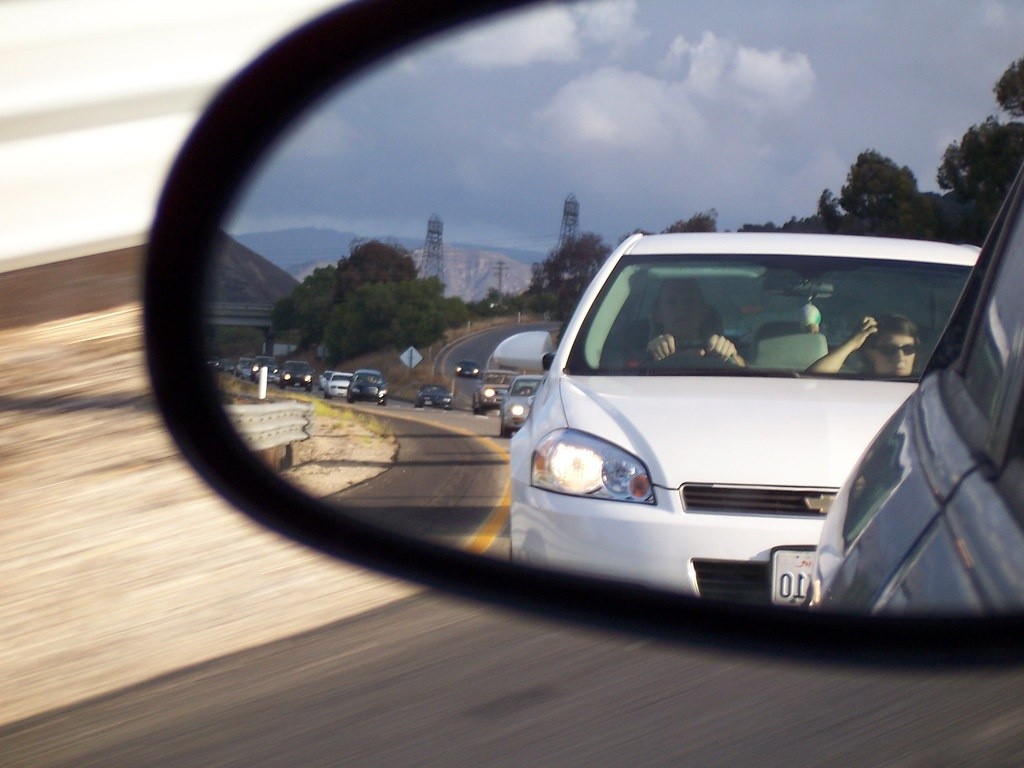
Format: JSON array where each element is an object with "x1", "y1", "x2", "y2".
[{"x1": 746, "y1": 322, "x2": 830, "y2": 370}]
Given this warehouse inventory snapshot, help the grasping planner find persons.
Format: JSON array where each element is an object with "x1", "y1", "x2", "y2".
[
  {"x1": 624, "y1": 291, "x2": 746, "y2": 368},
  {"x1": 805, "y1": 314, "x2": 921, "y2": 379}
]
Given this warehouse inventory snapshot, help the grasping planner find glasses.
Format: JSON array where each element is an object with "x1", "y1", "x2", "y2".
[{"x1": 872, "y1": 344, "x2": 916, "y2": 355}]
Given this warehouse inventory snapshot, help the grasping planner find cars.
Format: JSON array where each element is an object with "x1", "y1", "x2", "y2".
[
  {"x1": 235, "y1": 358, "x2": 254, "y2": 376},
  {"x1": 251, "y1": 356, "x2": 282, "y2": 384},
  {"x1": 812, "y1": 160, "x2": 1023, "y2": 611},
  {"x1": 415, "y1": 384, "x2": 452, "y2": 411},
  {"x1": 324, "y1": 372, "x2": 354, "y2": 399},
  {"x1": 496, "y1": 375, "x2": 545, "y2": 439},
  {"x1": 347, "y1": 370, "x2": 389, "y2": 406},
  {"x1": 473, "y1": 370, "x2": 521, "y2": 415},
  {"x1": 491, "y1": 232, "x2": 983, "y2": 606},
  {"x1": 456, "y1": 360, "x2": 479, "y2": 378},
  {"x1": 319, "y1": 371, "x2": 341, "y2": 390},
  {"x1": 279, "y1": 361, "x2": 316, "y2": 392}
]
